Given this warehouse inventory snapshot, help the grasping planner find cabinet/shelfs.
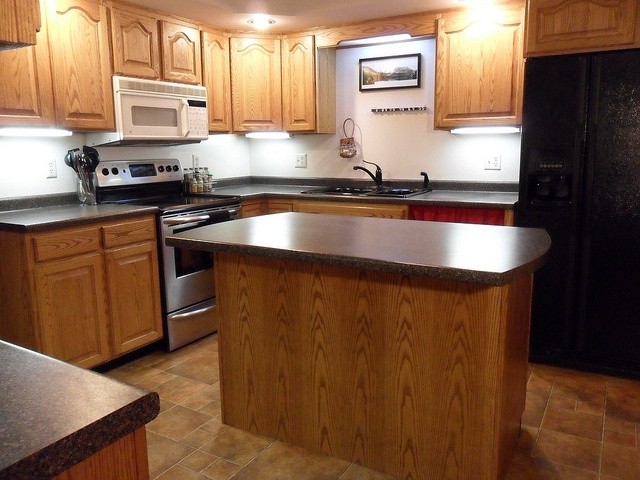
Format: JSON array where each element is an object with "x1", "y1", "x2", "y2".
[
  {"x1": 111, "y1": 6, "x2": 201, "y2": 83},
  {"x1": 230, "y1": 35, "x2": 281, "y2": 132},
  {"x1": 523, "y1": 0, "x2": 640, "y2": 56},
  {"x1": 1, "y1": 216, "x2": 162, "y2": 372},
  {"x1": 200, "y1": 30, "x2": 231, "y2": 131},
  {"x1": 0, "y1": 1, "x2": 115, "y2": 131},
  {"x1": 435, "y1": 14, "x2": 522, "y2": 129},
  {"x1": 283, "y1": 36, "x2": 317, "y2": 132}
]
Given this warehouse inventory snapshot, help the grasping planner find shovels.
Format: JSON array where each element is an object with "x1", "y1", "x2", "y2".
[
  {"x1": 85, "y1": 152, "x2": 99, "y2": 196},
  {"x1": 82, "y1": 145, "x2": 99, "y2": 155}
]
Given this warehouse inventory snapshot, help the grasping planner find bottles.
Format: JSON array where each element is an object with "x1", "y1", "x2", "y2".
[
  {"x1": 195, "y1": 168, "x2": 199, "y2": 177},
  {"x1": 208, "y1": 173, "x2": 213, "y2": 190},
  {"x1": 198, "y1": 174, "x2": 203, "y2": 193},
  {"x1": 191, "y1": 172, "x2": 198, "y2": 194},
  {"x1": 204, "y1": 167, "x2": 209, "y2": 176},
  {"x1": 183, "y1": 168, "x2": 190, "y2": 196},
  {"x1": 199, "y1": 167, "x2": 204, "y2": 176},
  {"x1": 204, "y1": 175, "x2": 208, "y2": 192},
  {"x1": 189, "y1": 167, "x2": 194, "y2": 181}
]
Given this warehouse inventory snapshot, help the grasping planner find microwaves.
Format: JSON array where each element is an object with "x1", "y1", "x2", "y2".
[{"x1": 84, "y1": 75, "x2": 209, "y2": 148}]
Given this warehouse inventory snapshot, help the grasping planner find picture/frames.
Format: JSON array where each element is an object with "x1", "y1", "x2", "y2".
[{"x1": 358, "y1": 53, "x2": 422, "y2": 91}]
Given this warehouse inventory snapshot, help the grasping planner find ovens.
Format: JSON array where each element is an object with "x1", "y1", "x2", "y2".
[{"x1": 90, "y1": 158, "x2": 243, "y2": 318}]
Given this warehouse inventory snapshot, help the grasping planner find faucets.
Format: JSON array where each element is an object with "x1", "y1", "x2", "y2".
[{"x1": 354, "y1": 159, "x2": 382, "y2": 187}]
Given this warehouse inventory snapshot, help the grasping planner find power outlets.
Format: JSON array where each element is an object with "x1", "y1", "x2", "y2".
[
  {"x1": 294, "y1": 153, "x2": 307, "y2": 168},
  {"x1": 484, "y1": 155, "x2": 501, "y2": 170}
]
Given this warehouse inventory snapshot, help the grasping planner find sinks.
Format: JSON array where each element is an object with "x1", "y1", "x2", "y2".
[
  {"x1": 375, "y1": 188, "x2": 417, "y2": 197},
  {"x1": 309, "y1": 185, "x2": 373, "y2": 195}
]
